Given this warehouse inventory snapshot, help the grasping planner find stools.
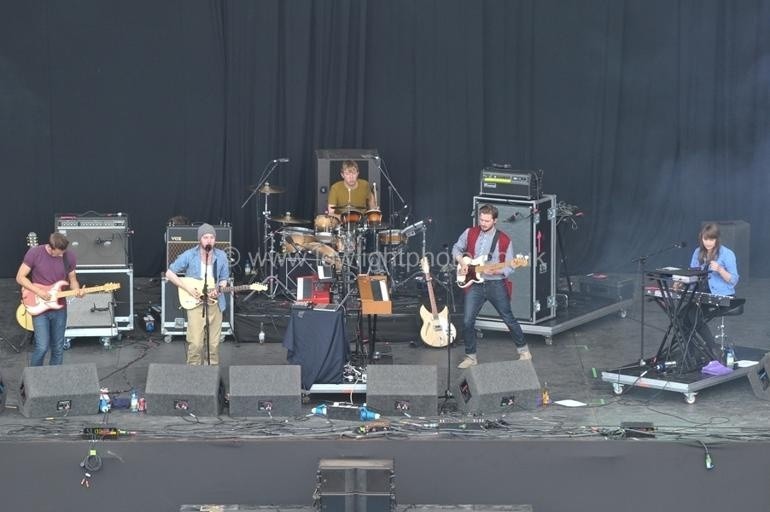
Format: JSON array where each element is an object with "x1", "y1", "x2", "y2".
[{"x1": 706, "y1": 299, "x2": 744, "y2": 367}]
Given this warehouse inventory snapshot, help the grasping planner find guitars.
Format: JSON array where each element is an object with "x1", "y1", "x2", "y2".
[
  {"x1": 20, "y1": 281, "x2": 121, "y2": 314},
  {"x1": 419, "y1": 258, "x2": 457, "y2": 347},
  {"x1": 17, "y1": 231, "x2": 42, "y2": 333},
  {"x1": 177, "y1": 277, "x2": 268, "y2": 308},
  {"x1": 457, "y1": 255, "x2": 526, "y2": 288}
]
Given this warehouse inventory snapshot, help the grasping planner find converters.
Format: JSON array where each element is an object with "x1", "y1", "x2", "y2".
[{"x1": 620, "y1": 421, "x2": 654, "y2": 439}]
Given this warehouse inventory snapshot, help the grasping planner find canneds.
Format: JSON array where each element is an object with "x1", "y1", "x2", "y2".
[{"x1": 138, "y1": 398, "x2": 145, "y2": 412}]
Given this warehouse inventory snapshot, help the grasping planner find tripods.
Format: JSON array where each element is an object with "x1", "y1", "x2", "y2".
[{"x1": 605, "y1": 260, "x2": 667, "y2": 377}]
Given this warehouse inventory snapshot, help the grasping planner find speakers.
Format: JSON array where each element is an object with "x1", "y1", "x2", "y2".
[
  {"x1": 229, "y1": 364, "x2": 303, "y2": 418},
  {"x1": 145, "y1": 363, "x2": 226, "y2": 417},
  {"x1": 453, "y1": 360, "x2": 541, "y2": 415},
  {"x1": 367, "y1": 364, "x2": 438, "y2": 417},
  {"x1": 478, "y1": 167, "x2": 545, "y2": 201},
  {"x1": 701, "y1": 219, "x2": 750, "y2": 288},
  {"x1": 16, "y1": 362, "x2": 100, "y2": 419}
]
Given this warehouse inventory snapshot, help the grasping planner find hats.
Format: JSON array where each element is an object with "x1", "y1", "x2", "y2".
[{"x1": 198, "y1": 223, "x2": 216, "y2": 240}]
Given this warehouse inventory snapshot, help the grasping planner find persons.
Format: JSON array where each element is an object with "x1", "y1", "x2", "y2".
[
  {"x1": 673, "y1": 223, "x2": 740, "y2": 360},
  {"x1": 16, "y1": 232, "x2": 86, "y2": 366},
  {"x1": 328, "y1": 159, "x2": 376, "y2": 215},
  {"x1": 451, "y1": 204, "x2": 533, "y2": 369},
  {"x1": 165, "y1": 223, "x2": 230, "y2": 366}
]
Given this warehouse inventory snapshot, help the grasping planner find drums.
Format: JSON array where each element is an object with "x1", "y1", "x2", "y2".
[
  {"x1": 341, "y1": 210, "x2": 361, "y2": 226},
  {"x1": 379, "y1": 230, "x2": 402, "y2": 248},
  {"x1": 333, "y1": 233, "x2": 345, "y2": 251},
  {"x1": 314, "y1": 216, "x2": 339, "y2": 231},
  {"x1": 366, "y1": 210, "x2": 380, "y2": 224},
  {"x1": 280, "y1": 226, "x2": 315, "y2": 253},
  {"x1": 290, "y1": 242, "x2": 342, "y2": 275},
  {"x1": 316, "y1": 232, "x2": 333, "y2": 243}
]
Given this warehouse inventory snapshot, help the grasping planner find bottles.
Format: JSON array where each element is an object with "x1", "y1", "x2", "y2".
[
  {"x1": 244, "y1": 260, "x2": 251, "y2": 272},
  {"x1": 258, "y1": 322, "x2": 265, "y2": 345},
  {"x1": 724, "y1": 343, "x2": 734, "y2": 367},
  {"x1": 131, "y1": 389, "x2": 145, "y2": 412},
  {"x1": 543, "y1": 381, "x2": 549, "y2": 404},
  {"x1": 145, "y1": 300, "x2": 153, "y2": 317}
]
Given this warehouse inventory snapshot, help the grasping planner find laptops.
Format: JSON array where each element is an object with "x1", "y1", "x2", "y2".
[{"x1": 312, "y1": 288, "x2": 355, "y2": 312}]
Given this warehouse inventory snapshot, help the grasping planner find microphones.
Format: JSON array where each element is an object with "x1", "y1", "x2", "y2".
[
  {"x1": 360, "y1": 153, "x2": 379, "y2": 160},
  {"x1": 399, "y1": 217, "x2": 433, "y2": 238},
  {"x1": 205, "y1": 244, "x2": 212, "y2": 256},
  {"x1": 673, "y1": 240, "x2": 688, "y2": 250},
  {"x1": 272, "y1": 158, "x2": 289, "y2": 164}
]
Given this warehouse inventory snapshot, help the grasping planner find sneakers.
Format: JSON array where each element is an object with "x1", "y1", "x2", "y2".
[
  {"x1": 458, "y1": 353, "x2": 477, "y2": 369},
  {"x1": 519, "y1": 351, "x2": 532, "y2": 360}
]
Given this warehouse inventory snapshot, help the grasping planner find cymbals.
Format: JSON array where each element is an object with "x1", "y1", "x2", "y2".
[
  {"x1": 250, "y1": 186, "x2": 284, "y2": 195},
  {"x1": 267, "y1": 214, "x2": 311, "y2": 225}
]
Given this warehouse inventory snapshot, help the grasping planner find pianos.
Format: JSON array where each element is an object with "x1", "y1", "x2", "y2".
[{"x1": 645, "y1": 282, "x2": 745, "y2": 309}]
